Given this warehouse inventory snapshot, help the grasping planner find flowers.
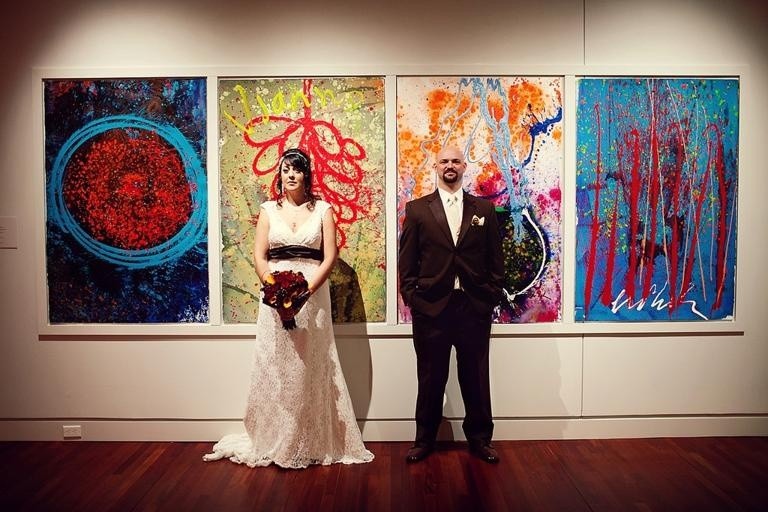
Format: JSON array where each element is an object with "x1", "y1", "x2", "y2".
[{"x1": 260, "y1": 270, "x2": 310, "y2": 330}]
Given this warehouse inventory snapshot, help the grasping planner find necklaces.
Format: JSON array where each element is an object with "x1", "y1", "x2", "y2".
[{"x1": 283, "y1": 199, "x2": 307, "y2": 228}]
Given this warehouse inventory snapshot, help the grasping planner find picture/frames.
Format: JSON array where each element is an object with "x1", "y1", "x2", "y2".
[{"x1": 31, "y1": 64, "x2": 753, "y2": 338}]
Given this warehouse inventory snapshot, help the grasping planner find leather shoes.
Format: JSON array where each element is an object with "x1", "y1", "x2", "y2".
[
  {"x1": 469, "y1": 440, "x2": 499, "y2": 462},
  {"x1": 406, "y1": 444, "x2": 433, "y2": 462}
]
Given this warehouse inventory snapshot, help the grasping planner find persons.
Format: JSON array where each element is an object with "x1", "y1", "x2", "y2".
[
  {"x1": 397, "y1": 146, "x2": 507, "y2": 465},
  {"x1": 200, "y1": 148, "x2": 377, "y2": 470}
]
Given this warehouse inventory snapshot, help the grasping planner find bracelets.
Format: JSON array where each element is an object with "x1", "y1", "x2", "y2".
[{"x1": 260, "y1": 269, "x2": 271, "y2": 279}]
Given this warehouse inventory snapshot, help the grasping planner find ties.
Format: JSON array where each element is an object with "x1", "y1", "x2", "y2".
[{"x1": 446, "y1": 197, "x2": 460, "y2": 238}]
